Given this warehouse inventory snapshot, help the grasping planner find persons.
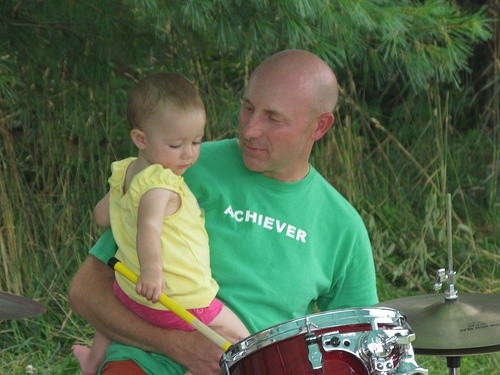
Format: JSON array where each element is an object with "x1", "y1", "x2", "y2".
[
  {"x1": 72, "y1": 74, "x2": 254, "y2": 375},
  {"x1": 66, "y1": 49, "x2": 380, "y2": 375}
]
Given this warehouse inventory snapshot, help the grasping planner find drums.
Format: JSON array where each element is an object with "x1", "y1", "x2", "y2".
[{"x1": 219, "y1": 304, "x2": 429, "y2": 375}]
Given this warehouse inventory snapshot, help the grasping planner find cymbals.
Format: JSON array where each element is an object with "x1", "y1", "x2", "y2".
[
  {"x1": 0, "y1": 289, "x2": 48, "y2": 323},
  {"x1": 371, "y1": 287, "x2": 500, "y2": 359}
]
